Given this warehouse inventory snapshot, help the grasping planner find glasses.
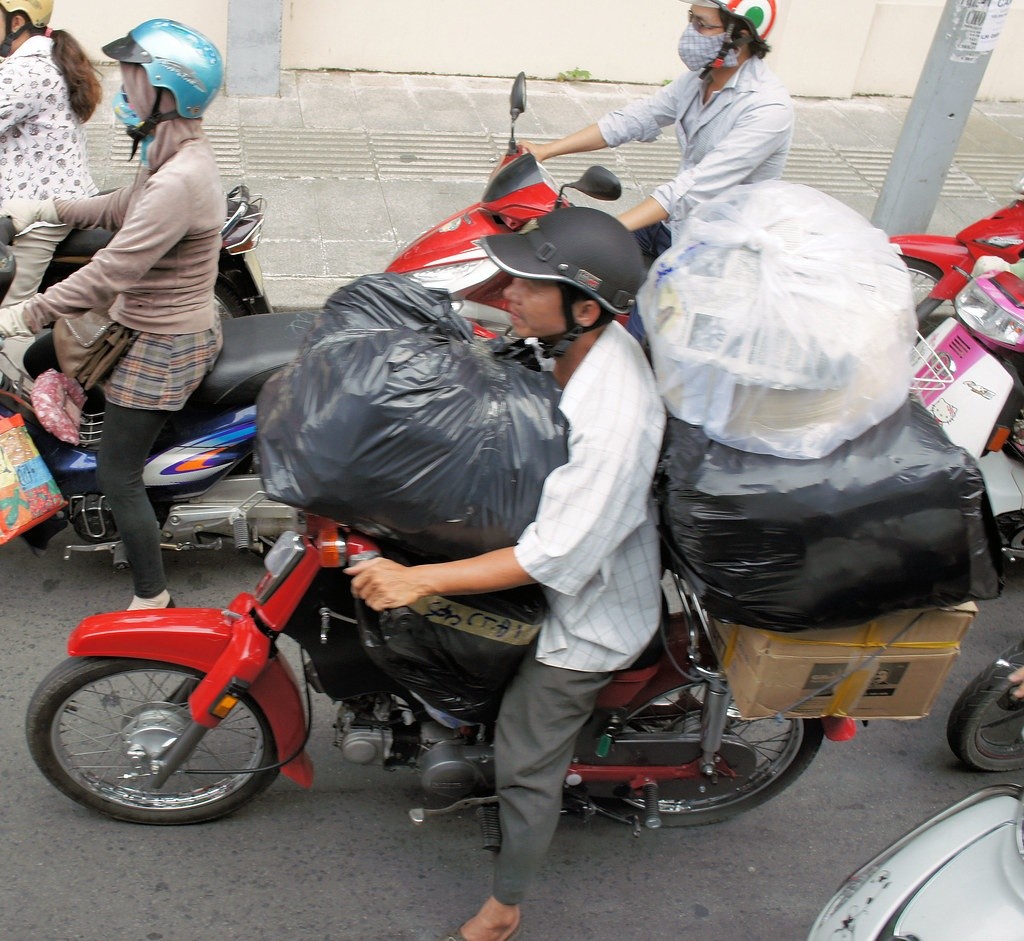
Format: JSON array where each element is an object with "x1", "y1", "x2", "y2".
[
  {"x1": 687, "y1": 11, "x2": 726, "y2": 31},
  {"x1": 120, "y1": 83, "x2": 130, "y2": 104}
]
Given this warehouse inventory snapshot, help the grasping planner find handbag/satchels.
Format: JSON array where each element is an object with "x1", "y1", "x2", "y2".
[
  {"x1": 0, "y1": 414, "x2": 69, "y2": 545},
  {"x1": 53, "y1": 305, "x2": 132, "y2": 390}
]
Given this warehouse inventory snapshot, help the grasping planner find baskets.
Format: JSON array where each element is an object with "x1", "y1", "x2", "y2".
[{"x1": 908, "y1": 328, "x2": 954, "y2": 408}]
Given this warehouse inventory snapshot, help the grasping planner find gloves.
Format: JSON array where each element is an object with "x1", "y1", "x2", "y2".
[
  {"x1": 0, "y1": 197, "x2": 62, "y2": 233},
  {"x1": 0, "y1": 300, "x2": 34, "y2": 337}
]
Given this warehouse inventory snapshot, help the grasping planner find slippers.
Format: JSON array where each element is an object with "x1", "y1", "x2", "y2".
[
  {"x1": 442, "y1": 908, "x2": 522, "y2": 941},
  {"x1": 164, "y1": 596, "x2": 177, "y2": 609}
]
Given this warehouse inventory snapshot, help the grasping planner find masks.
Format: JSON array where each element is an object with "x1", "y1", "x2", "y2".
[{"x1": 678, "y1": 26, "x2": 742, "y2": 72}]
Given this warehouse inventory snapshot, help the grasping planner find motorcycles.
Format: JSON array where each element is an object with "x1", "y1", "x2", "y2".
[
  {"x1": 389, "y1": 73, "x2": 635, "y2": 365},
  {"x1": 888, "y1": 201, "x2": 1024, "y2": 579},
  {"x1": 3, "y1": 183, "x2": 272, "y2": 318},
  {"x1": 944, "y1": 641, "x2": 1024, "y2": 775},
  {"x1": 24, "y1": 510, "x2": 857, "y2": 832},
  {"x1": 1, "y1": 220, "x2": 325, "y2": 552}
]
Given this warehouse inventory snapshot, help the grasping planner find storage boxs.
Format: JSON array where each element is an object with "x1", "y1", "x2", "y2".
[{"x1": 707, "y1": 601, "x2": 981, "y2": 725}]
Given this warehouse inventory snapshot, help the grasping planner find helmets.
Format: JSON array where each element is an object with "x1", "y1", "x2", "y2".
[
  {"x1": 0, "y1": 0, "x2": 54, "y2": 28},
  {"x1": 103, "y1": 17, "x2": 223, "y2": 118},
  {"x1": 681, "y1": 0, "x2": 777, "y2": 42},
  {"x1": 483, "y1": 207, "x2": 646, "y2": 314}
]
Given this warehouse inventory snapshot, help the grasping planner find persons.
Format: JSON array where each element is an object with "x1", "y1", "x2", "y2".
[
  {"x1": 0, "y1": 19, "x2": 225, "y2": 611},
  {"x1": 970, "y1": 256, "x2": 1024, "y2": 279},
  {"x1": 0, "y1": 0, "x2": 100, "y2": 386},
  {"x1": 343, "y1": 206, "x2": 664, "y2": 941},
  {"x1": 517, "y1": 0, "x2": 795, "y2": 253}
]
